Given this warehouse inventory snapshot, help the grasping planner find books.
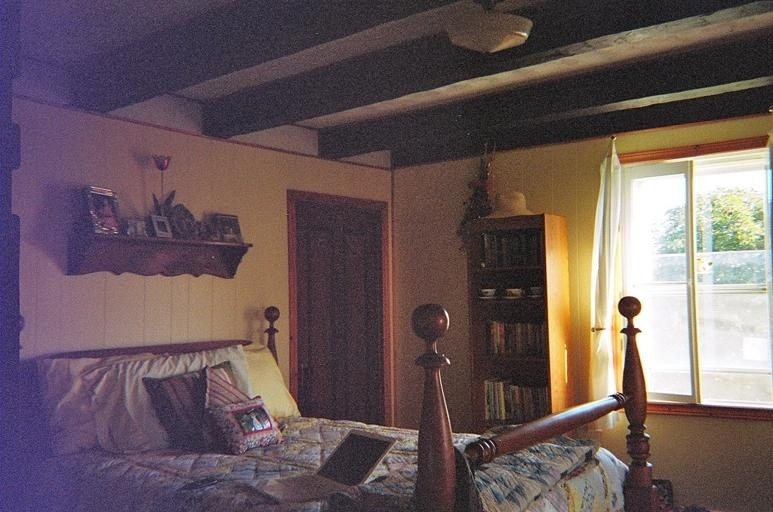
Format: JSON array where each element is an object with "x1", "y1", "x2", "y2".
[
  {"x1": 484, "y1": 376, "x2": 550, "y2": 421},
  {"x1": 483, "y1": 233, "x2": 542, "y2": 265},
  {"x1": 477, "y1": 316, "x2": 546, "y2": 355}
]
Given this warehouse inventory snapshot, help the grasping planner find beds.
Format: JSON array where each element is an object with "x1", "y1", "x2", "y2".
[{"x1": 17, "y1": 295, "x2": 658, "y2": 511}]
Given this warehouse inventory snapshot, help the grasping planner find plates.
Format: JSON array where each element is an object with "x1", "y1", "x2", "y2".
[
  {"x1": 527, "y1": 294, "x2": 544, "y2": 298},
  {"x1": 480, "y1": 297, "x2": 498, "y2": 300},
  {"x1": 504, "y1": 296, "x2": 523, "y2": 300}
]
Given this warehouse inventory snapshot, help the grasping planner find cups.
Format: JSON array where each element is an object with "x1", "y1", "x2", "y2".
[
  {"x1": 530, "y1": 287, "x2": 544, "y2": 296},
  {"x1": 478, "y1": 288, "x2": 496, "y2": 296},
  {"x1": 505, "y1": 288, "x2": 524, "y2": 296}
]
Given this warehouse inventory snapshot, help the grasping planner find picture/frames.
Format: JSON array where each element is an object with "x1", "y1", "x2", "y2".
[
  {"x1": 82, "y1": 186, "x2": 121, "y2": 236},
  {"x1": 150, "y1": 215, "x2": 172, "y2": 239}
]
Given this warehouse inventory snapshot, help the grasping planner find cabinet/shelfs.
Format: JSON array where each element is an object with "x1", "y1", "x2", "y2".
[{"x1": 465, "y1": 212, "x2": 574, "y2": 435}]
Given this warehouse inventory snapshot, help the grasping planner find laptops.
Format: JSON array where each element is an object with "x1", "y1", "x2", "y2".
[{"x1": 245, "y1": 429, "x2": 396, "y2": 502}]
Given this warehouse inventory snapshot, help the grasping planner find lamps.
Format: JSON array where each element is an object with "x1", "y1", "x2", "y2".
[{"x1": 443, "y1": 0, "x2": 533, "y2": 58}]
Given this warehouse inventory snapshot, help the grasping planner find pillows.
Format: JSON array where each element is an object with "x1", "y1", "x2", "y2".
[{"x1": 33, "y1": 341, "x2": 301, "y2": 458}]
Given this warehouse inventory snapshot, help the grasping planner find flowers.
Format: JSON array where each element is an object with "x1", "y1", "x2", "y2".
[{"x1": 150, "y1": 154, "x2": 175, "y2": 215}]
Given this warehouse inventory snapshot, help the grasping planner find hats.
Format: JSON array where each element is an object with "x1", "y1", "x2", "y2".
[{"x1": 478, "y1": 189, "x2": 538, "y2": 219}]
